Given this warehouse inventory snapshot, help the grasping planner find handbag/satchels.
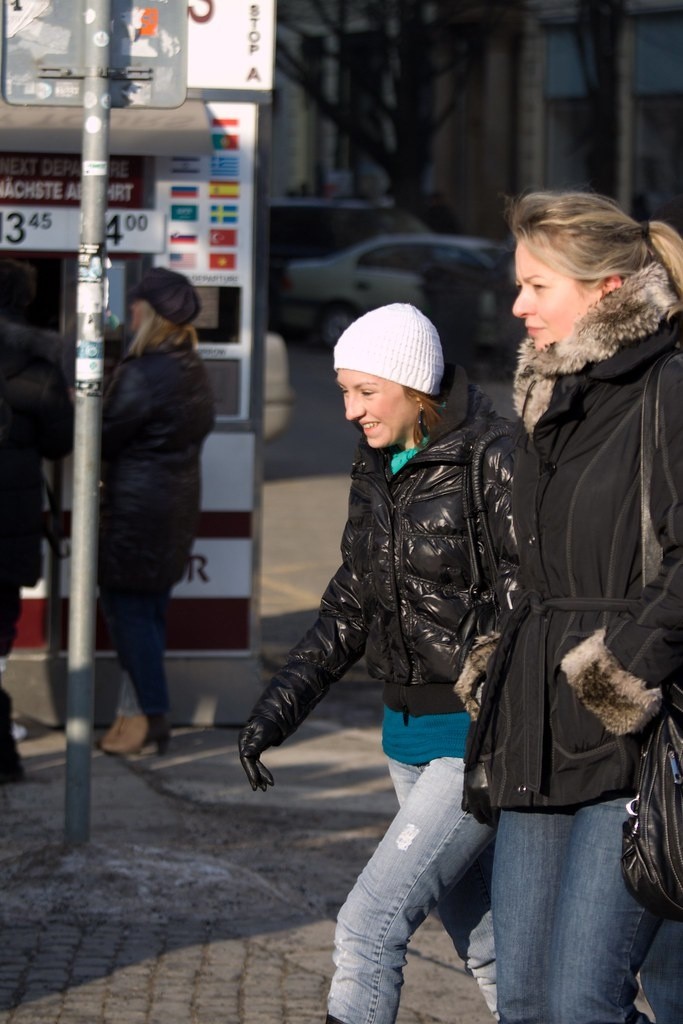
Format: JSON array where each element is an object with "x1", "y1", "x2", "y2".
[{"x1": 622, "y1": 715, "x2": 681, "y2": 930}]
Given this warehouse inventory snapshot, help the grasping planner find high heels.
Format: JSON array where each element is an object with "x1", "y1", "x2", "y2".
[{"x1": 103, "y1": 712, "x2": 158, "y2": 755}]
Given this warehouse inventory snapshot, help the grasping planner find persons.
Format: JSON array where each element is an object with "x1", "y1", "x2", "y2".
[
  {"x1": 0, "y1": 261, "x2": 71, "y2": 781},
  {"x1": 100, "y1": 669, "x2": 152, "y2": 756},
  {"x1": 452, "y1": 187, "x2": 682, "y2": 1024},
  {"x1": 239, "y1": 303, "x2": 521, "y2": 1023},
  {"x1": 99, "y1": 266, "x2": 210, "y2": 760}
]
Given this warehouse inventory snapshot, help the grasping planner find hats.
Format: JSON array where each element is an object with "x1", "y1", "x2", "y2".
[
  {"x1": 141, "y1": 268, "x2": 203, "y2": 328},
  {"x1": 333, "y1": 303, "x2": 445, "y2": 393}
]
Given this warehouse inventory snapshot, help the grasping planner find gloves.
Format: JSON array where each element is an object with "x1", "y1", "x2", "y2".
[{"x1": 237, "y1": 701, "x2": 286, "y2": 793}]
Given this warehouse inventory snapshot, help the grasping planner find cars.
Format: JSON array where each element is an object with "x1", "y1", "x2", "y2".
[{"x1": 281, "y1": 235, "x2": 509, "y2": 353}]
